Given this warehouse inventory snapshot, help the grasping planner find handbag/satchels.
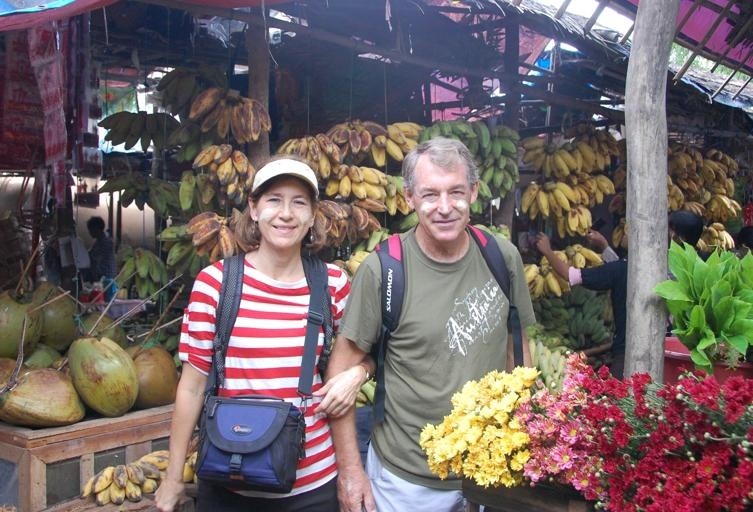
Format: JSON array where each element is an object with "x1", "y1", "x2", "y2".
[{"x1": 191, "y1": 393, "x2": 307, "y2": 495}]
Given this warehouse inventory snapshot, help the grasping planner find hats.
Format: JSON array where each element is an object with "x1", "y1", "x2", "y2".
[{"x1": 250, "y1": 156, "x2": 321, "y2": 200}]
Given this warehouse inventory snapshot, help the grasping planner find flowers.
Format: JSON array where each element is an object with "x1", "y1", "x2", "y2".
[{"x1": 416, "y1": 347, "x2": 753, "y2": 511}]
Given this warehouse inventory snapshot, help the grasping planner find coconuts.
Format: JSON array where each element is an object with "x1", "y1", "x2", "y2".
[{"x1": 0, "y1": 282, "x2": 179, "y2": 429}]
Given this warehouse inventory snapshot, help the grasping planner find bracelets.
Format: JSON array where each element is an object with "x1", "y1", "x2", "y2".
[{"x1": 357, "y1": 362, "x2": 374, "y2": 380}]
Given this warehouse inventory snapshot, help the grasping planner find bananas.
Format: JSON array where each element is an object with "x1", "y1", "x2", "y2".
[
  {"x1": 275, "y1": 118, "x2": 521, "y2": 409},
  {"x1": 519, "y1": 121, "x2": 753, "y2": 398},
  {"x1": 80, "y1": 436, "x2": 199, "y2": 506},
  {"x1": 97, "y1": 58, "x2": 272, "y2": 371}
]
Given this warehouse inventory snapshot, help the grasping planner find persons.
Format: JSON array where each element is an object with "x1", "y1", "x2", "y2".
[
  {"x1": 319, "y1": 132, "x2": 541, "y2": 511},
  {"x1": 666, "y1": 208, "x2": 715, "y2": 264},
  {"x1": 535, "y1": 217, "x2": 679, "y2": 384},
  {"x1": 150, "y1": 152, "x2": 378, "y2": 511},
  {"x1": 77, "y1": 215, "x2": 115, "y2": 304}
]
